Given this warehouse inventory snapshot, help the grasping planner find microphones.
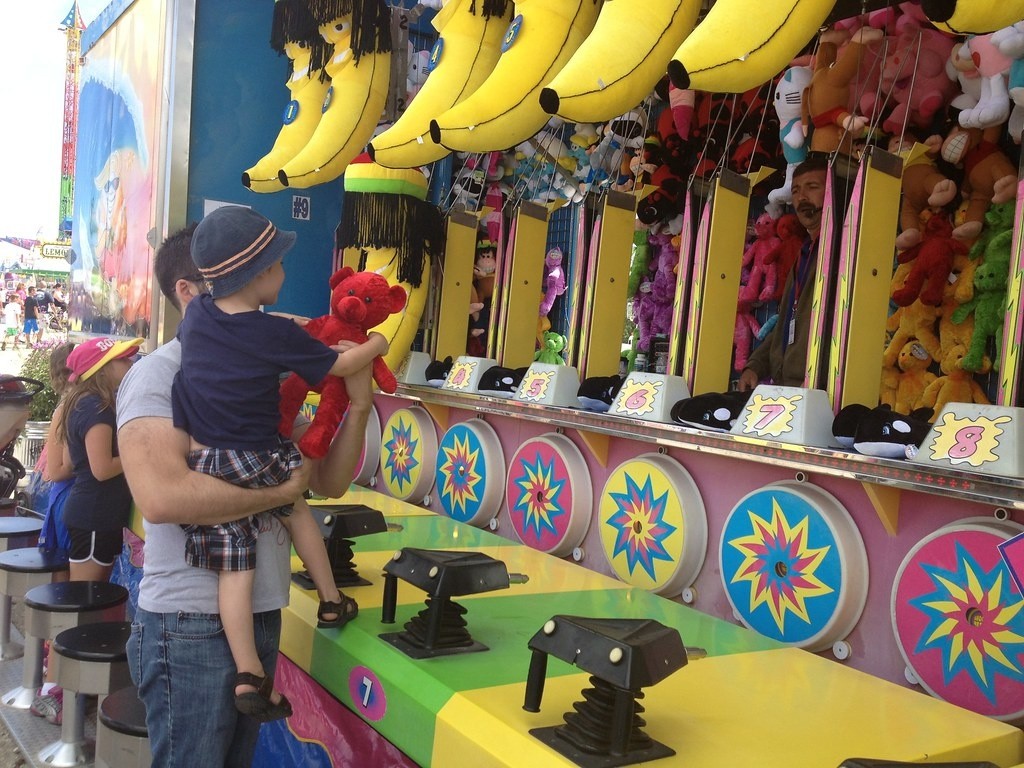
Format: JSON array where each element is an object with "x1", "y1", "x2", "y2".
[{"x1": 806, "y1": 206, "x2": 823, "y2": 218}]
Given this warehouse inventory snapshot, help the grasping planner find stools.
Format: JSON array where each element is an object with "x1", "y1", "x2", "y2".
[{"x1": 0, "y1": 493, "x2": 154, "y2": 768}]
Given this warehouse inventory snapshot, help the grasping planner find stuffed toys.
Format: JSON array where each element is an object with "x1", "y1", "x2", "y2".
[
  {"x1": 242, "y1": 0, "x2": 530, "y2": 398},
  {"x1": 730, "y1": 0, "x2": 1024, "y2": 459},
  {"x1": 515, "y1": 1, "x2": 753, "y2": 433},
  {"x1": 278, "y1": 266, "x2": 407, "y2": 459}
]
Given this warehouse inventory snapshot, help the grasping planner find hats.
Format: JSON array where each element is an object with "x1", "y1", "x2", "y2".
[
  {"x1": 66, "y1": 337, "x2": 144, "y2": 382},
  {"x1": 189, "y1": 205, "x2": 296, "y2": 300}
]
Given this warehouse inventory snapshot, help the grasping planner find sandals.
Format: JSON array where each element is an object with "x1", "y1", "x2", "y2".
[
  {"x1": 317, "y1": 590, "x2": 358, "y2": 629},
  {"x1": 231, "y1": 672, "x2": 293, "y2": 722}
]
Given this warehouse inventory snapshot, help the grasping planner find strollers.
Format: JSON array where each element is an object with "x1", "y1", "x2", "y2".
[{"x1": 0, "y1": 373, "x2": 45, "y2": 516}]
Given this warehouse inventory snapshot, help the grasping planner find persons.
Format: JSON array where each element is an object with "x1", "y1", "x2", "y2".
[
  {"x1": 1, "y1": 281, "x2": 67, "y2": 352},
  {"x1": 172, "y1": 205, "x2": 389, "y2": 722},
  {"x1": 30, "y1": 337, "x2": 144, "y2": 725},
  {"x1": 738, "y1": 159, "x2": 828, "y2": 395},
  {"x1": 116, "y1": 221, "x2": 374, "y2": 768}
]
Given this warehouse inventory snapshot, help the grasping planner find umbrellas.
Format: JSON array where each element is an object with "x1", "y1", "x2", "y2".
[{"x1": 9, "y1": 256, "x2": 70, "y2": 279}]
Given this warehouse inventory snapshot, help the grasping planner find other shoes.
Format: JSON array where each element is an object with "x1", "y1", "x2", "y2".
[
  {"x1": 1, "y1": 341, "x2": 6, "y2": 350},
  {"x1": 30, "y1": 686, "x2": 63, "y2": 726},
  {"x1": 14, "y1": 344, "x2": 19, "y2": 349},
  {"x1": 42, "y1": 643, "x2": 49, "y2": 674}
]
